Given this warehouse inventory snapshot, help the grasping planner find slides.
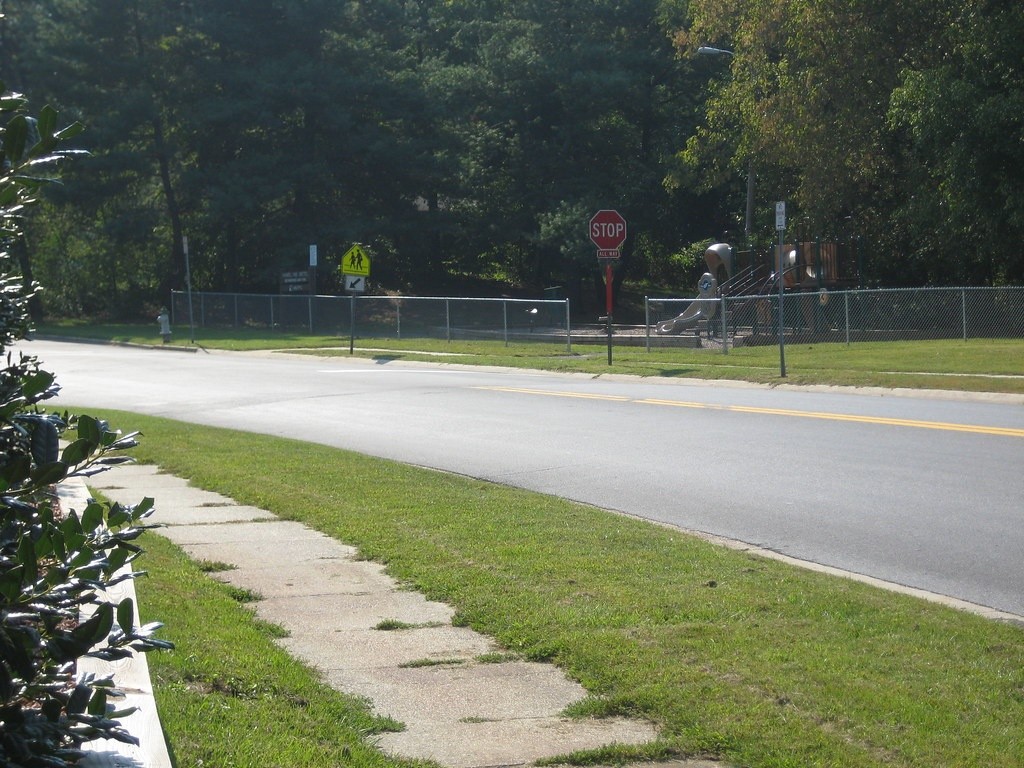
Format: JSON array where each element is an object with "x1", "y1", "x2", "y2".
[{"x1": 657, "y1": 293, "x2": 718, "y2": 335}]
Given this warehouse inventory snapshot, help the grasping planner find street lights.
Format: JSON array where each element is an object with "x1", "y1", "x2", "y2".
[{"x1": 697, "y1": 46, "x2": 754, "y2": 245}]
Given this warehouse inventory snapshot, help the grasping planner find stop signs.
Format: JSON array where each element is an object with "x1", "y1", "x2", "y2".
[{"x1": 589, "y1": 209, "x2": 627, "y2": 251}]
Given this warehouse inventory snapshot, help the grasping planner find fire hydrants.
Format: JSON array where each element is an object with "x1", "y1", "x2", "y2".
[{"x1": 157, "y1": 305, "x2": 172, "y2": 343}]
